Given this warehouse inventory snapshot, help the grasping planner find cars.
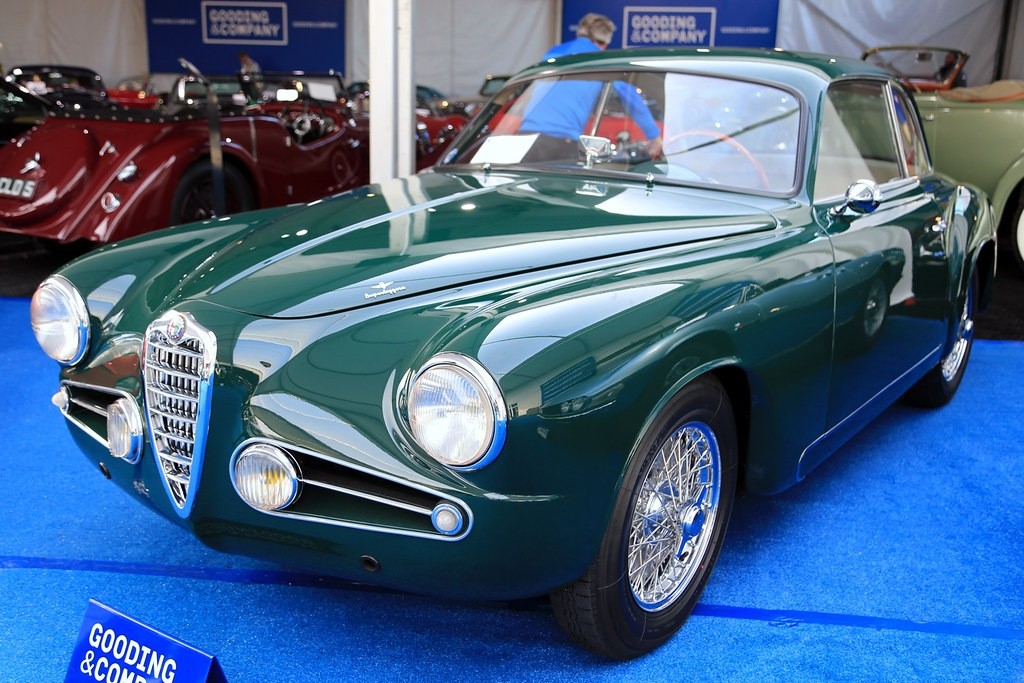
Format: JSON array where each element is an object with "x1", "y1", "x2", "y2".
[
  {"x1": 26, "y1": 41, "x2": 999, "y2": 664},
  {"x1": 0, "y1": 44, "x2": 1024, "y2": 289}
]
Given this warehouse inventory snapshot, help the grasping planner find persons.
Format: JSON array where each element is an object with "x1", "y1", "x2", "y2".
[
  {"x1": 519, "y1": 14, "x2": 663, "y2": 161},
  {"x1": 240, "y1": 52, "x2": 261, "y2": 74},
  {"x1": 69, "y1": 80, "x2": 86, "y2": 91},
  {"x1": 26, "y1": 73, "x2": 46, "y2": 94}
]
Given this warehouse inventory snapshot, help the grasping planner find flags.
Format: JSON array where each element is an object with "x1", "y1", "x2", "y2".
[
  {"x1": 145, "y1": 0, "x2": 346, "y2": 79},
  {"x1": 562, "y1": 0, "x2": 779, "y2": 105}
]
